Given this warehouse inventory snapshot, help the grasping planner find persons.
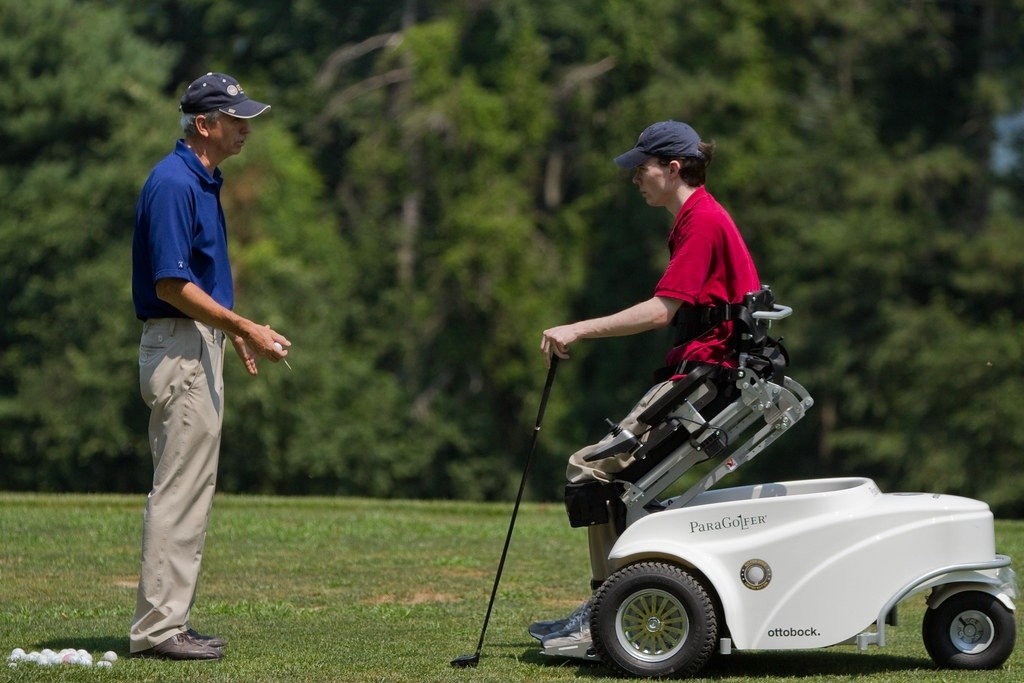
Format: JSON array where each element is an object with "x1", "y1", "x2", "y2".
[
  {"x1": 528, "y1": 119, "x2": 791, "y2": 654},
  {"x1": 129, "y1": 72, "x2": 292, "y2": 660}
]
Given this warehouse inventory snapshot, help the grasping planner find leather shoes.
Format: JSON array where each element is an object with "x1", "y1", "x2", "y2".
[{"x1": 134, "y1": 626, "x2": 230, "y2": 660}]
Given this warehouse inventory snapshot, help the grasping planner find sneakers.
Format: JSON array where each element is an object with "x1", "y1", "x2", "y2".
[{"x1": 528, "y1": 581, "x2": 602, "y2": 652}]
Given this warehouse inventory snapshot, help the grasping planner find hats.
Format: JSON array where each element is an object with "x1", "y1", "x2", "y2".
[
  {"x1": 613, "y1": 120, "x2": 701, "y2": 164},
  {"x1": 180, "y1": 73, "x2": 271, "y2": 118}
]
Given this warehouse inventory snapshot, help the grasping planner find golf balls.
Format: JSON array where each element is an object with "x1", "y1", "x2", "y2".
[
  {"x1": 272, "y1": 340, "x2": 284, "y2": 352},
  {"x1": 7, "y1": 645, "x2": 119, "y2": 669}
]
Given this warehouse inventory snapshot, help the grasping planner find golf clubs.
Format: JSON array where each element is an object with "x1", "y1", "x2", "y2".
[{"x1": 449, "y1": 347, "x2": 560, "y2": 669}]
